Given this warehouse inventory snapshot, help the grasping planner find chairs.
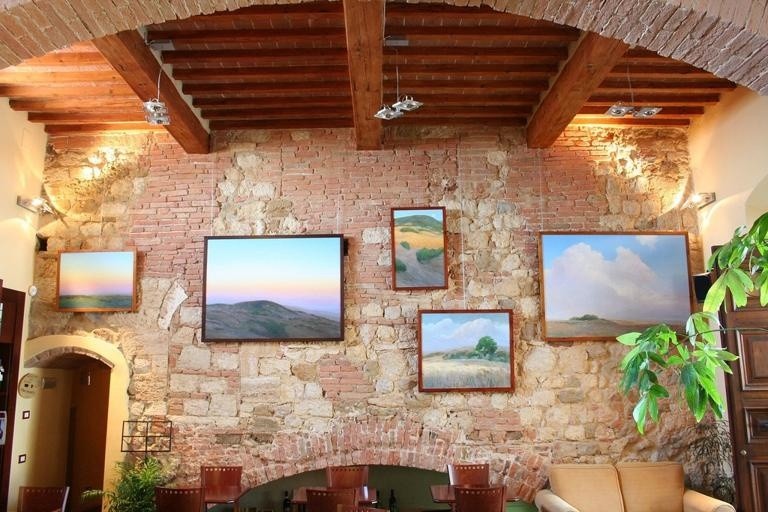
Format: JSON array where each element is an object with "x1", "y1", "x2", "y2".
[
  {"x1": 446, "y1": 464, "x2": 491, "y2": 486},
  {"x1": 453, "y1": 485, "x2": 506, "y2": 511},
  {"x1": 153, "y1": 485, "x2": 205, "y2": 511},
  {"x1": 17, "y1": 485, "x2": 71, "y2": 511},
  {"x1": 198, "y1": 465, "x2": 248, "y2": 505},
  {"x1": 327, "y1": 464, "x2": 369, "y2": 488},
  {"x1": 305, "y1": 487, "x2": 360, "y2": 510}
]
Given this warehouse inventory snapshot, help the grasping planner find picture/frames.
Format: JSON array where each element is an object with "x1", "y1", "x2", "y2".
[
  {"x1": 537, "y1": 229, "x2": 693, "y2": 342},
  {"x1": 390, "y1": 206, "x2": 449, "y2": 293},
  {"x1": 53, "y1": 247, "x2": 139, "y2": 314},
  {"x1": 204, "y1": 231, "x2": 345, "y2": 344},
  {"x1": 415, "y1": 307, "x2": 518, "y2": 394}
]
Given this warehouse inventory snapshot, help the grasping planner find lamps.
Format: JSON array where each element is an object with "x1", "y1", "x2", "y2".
[
  {"x1": 140, "y1": 61, "x2": 172, "y2": 128},
  {"x1": 603, "y1": 66, "x2": 663, "y2": 120},
  {"x1": 373, "y1": 62, "x2": 424, "y2": 121}
]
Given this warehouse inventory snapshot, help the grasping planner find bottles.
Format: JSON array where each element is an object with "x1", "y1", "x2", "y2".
[
  {"x1": 375, "y1": 490, "x2": 398, "y2": 512},
  {"x1": 282, "y1": 491, "x2": 292, "y2": 511}
]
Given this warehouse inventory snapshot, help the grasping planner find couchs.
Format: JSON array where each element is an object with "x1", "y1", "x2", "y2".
[{"x1": 535, "y1": 458, "x2": 735, "y2": 512}]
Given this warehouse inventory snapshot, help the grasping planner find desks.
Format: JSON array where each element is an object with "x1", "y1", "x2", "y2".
[
  {"x1": 429, "y1": 483, "x2": 519, "y2": 511},
  {"x1": 170, "y1": 482, "x2": 249, "y2": 507},
  {"x1": 287, "y1": 486, "x2": 377, "y2": 512}
]
